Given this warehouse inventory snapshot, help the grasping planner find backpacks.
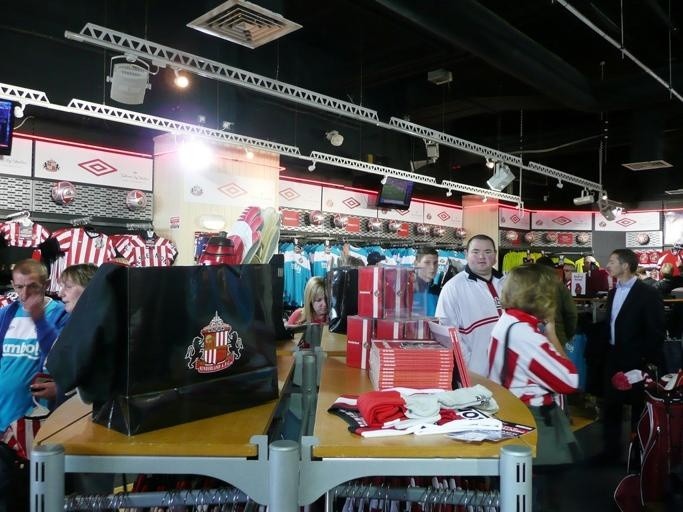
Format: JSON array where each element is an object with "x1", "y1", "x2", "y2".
[{"x1": 327, "y1": 267, "x2": 357, "y2": 335}]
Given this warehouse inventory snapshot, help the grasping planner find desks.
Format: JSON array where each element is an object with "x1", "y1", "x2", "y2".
[{"x1": 28, "y1": 323, "x2": 538, "y2": 511}]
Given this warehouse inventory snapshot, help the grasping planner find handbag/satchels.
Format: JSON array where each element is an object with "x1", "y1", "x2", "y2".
[{"x1": 92, "y1": 263, "x2": 278, "y2": 437}]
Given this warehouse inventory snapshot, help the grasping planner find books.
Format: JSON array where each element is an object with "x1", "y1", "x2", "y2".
[{"x1": 369, "y1": 321, "x2": 471, "y2": 392}]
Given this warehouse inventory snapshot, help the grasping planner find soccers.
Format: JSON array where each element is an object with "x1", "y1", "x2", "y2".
[
  {"x1": 525, "y1": 232, "x2": 535, "y2": 243},
  {"x1": 507, "y1": 231, "x2": 518, "y2": 241},
  {"x1": 368, "y1": 217, "x2": 383, "y2": 232},
  {"x1": 456, "y1": 228, "x2": 466, "y2": 238},
  {"x1": 547, "y1": 232, "x2": 558, "y2": 242},
  {"x1": 577, "y1": 232, "x2": 589, "y2": 243},
  {"x1": 334, "y1": 215, "x2": 348, "y2": 227},
  {"x1": 638, "y1": 234, "x2": 649, "y2": 245},
  {"x1": 417, "y1": 223, "x2": 430, "y2": 235},
  {"x1": 308, "y1": 211, "x2": 324, "y2": 225},
  {"x1": 126, "y1": 191, "x2": 147, "y2": 213},
  {"x1": 389, "y1": 220, "x2": 401, "y2": 232},
  {"x1": 52, "y1": 181, "x2": 75, "y2": 204},
  {"x1": 433, "y1": 225, "x2": 446, "y2": 238}
]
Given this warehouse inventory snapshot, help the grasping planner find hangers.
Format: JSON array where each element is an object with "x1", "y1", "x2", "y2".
[
  {"x1": 279, "y1": 233, "x2": 465, "y2": 252},
  {"x1": 71, "y1": 214, "x2": 100, "y2": 238},
  {"x1": 125, "y1": 220, "x2": 158, "y2": 244},
  {"x1": 504, "y1": 246, "x2": 592, "y2": 263},
  {"x1": 336, "y1": 477, "x2": 499, "y2": 511},
  {"x1": 6, "y1": 211, "x2": 37, "y2": 239},
  {"x1": 64, "y1": 486, "x2": 266, "y2": 512},
  {"x1": 633, "y1": 241, "x2": 681, "y2": 254}
]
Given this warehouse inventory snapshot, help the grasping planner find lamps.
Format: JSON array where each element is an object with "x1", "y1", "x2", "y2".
[
  {"x1": 486, "y1": 164, "x2": 516, "y2": 191},
  {"x1": 482, "y1": 195, "x2": 487, "y2": 203},
  {"x1": 221, "y1": 120, "x2": 234, "y2": 131},
  {"x1": 446, "y1": 188, "x2": 452, "y2": 197},
  {"x1": 326, "y1": 129, "x2": 344, "y2": 147},
  {"x1": 427, "y1": 68, "x2": 453, "y2": 85},
  {"x1": 380, "y1": 175, "x2": 388, "y2": 184},
  {"x1": 13, "y1": 104, "x2": 25, "y2": 118},
  {"x1": 171, "y1": 66, "x2": 188, "y2": 87},
  {"x1": 409, "y1": 144, "x2": 439, "y2": 172},
  {"x1": 308, "y1": 161, "x2": 316, "y2": 172},
  {"x1": 573, "y1": 188, "x2": 595, "y2": 206}
]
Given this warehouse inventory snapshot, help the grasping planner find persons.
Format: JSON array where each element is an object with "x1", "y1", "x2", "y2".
[
  {"x1": 577, "y1": 249, "x2": 666, "y2": 473},
  {"x1": 410, "y1": 248, "x2": 443, "y2": 322},
  {"x1": 536, "y1": 256, "x2": 578, "y2": 350},
  {"x1": 433, "y1": 234, "x2": 506, "y2": 380},
  {"x1": 0, "y1": 259, "x2": 72, "y2": 512},
  {"x1": 484, "y1": 263, "x2": 585, "y2": 512},
  {"x1": 24, "y1": 263, "x2": 99, "y2": 410},
  {"x1": 288, "y1": 276, "x2": 332, "y2": 325},
  {"x1": 563, "y1": 256, "x2": 683, "y2": 298}
]
